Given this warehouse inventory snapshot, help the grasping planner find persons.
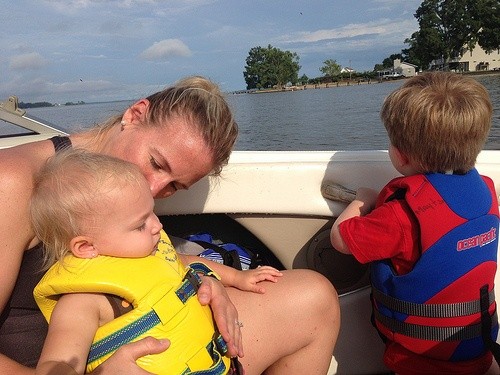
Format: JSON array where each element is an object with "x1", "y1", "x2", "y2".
[
  {"x1": 26, "y1": 145, "x2": 341, "y2": 375},
  {"x1": 0, "y1": 74, "x2": 240, "y2": 375},
  {"x1": 330, "y1": 71, "x2": 500, "y2": 375}
]
